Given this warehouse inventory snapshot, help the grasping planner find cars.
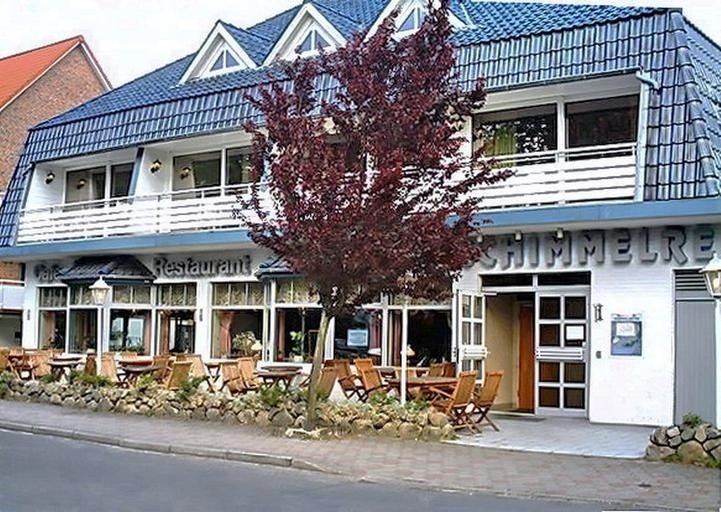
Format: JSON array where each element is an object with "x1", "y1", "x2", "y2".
[{"x1": 334, "y1": 336, "x2": 366, "y2": 359}]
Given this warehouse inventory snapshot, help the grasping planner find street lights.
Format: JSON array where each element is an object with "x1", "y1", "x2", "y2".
[{"x1": 87, "y1": 275, "x2": 113, "y2": 375}]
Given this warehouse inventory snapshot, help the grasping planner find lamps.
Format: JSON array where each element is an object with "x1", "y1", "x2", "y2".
[
  {"x1": 44, "y1": 168, "x2": 58, "y2": 185},
  {"x1": 77, "y1": 177, "x2": 87, "y2": 188},
  {"x1": 515, "y1": 228, "x2": 524, "y2": 241},
  {"x1": 178, "y1": 165, "x2": 192, "y2": 180},
  {"x1": 477, "y1": 231, "x2": 484, "y2": 243},
  {"x1": 150, "y1": 156, "x2": 163, "y2": 173},
  {"x1": 555, "y1": 226, "x2": 564, "y2": 239}
]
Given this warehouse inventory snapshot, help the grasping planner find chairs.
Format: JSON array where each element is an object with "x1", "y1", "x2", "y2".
[{"x1": 0, "y1": 342, "x2": 505, "y2": 440}]
